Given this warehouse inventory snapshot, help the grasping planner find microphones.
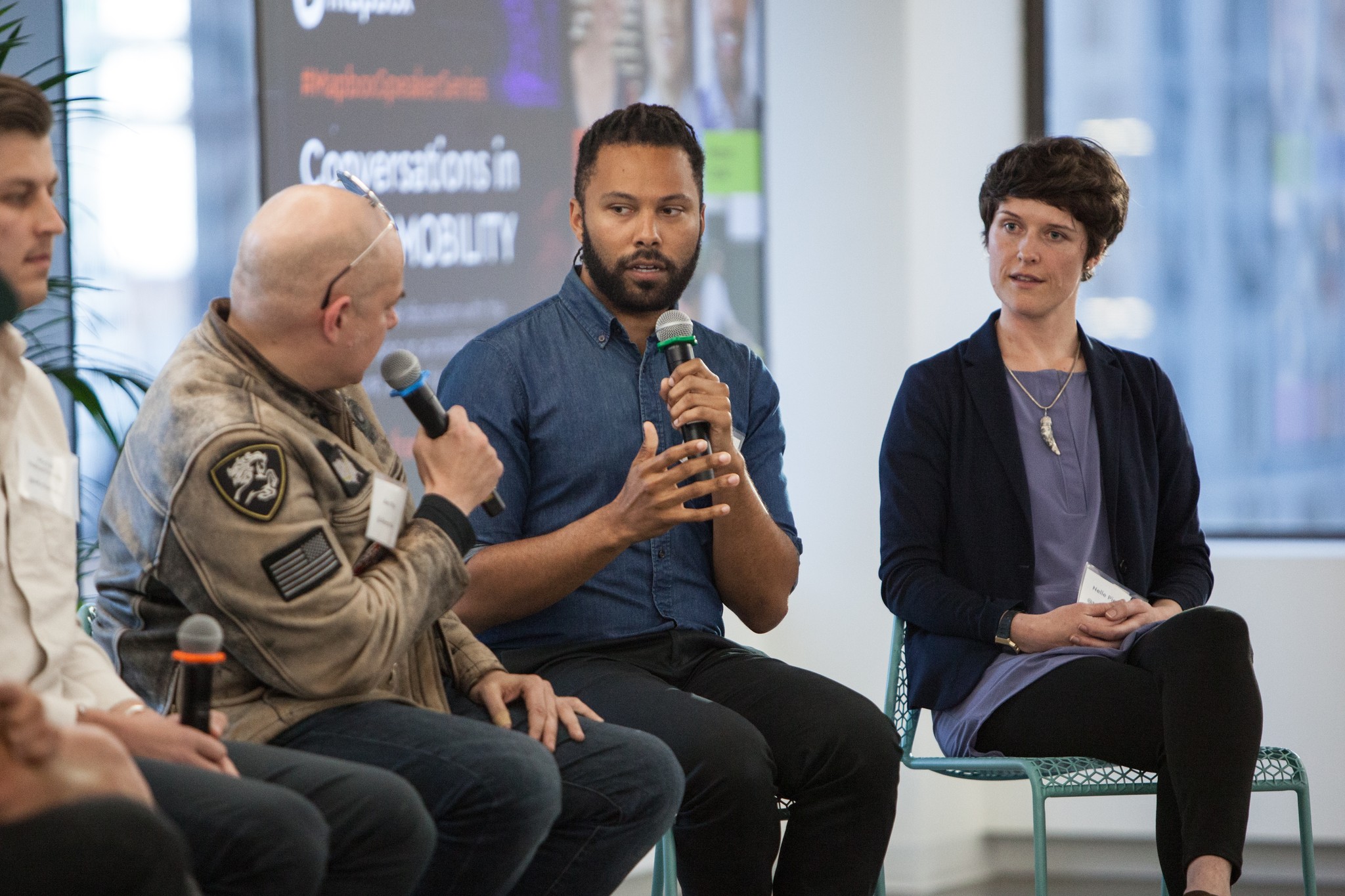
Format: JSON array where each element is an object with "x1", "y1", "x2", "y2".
[
  {"x1": 380, "y1": 349, "x2": 506, "y2": 518},
  {"x1": 656, "y1": 309, "x2": 715, "y2": 483},
  {"x1": 172, "y1": 612, "x2": 225, "y2": 735}
]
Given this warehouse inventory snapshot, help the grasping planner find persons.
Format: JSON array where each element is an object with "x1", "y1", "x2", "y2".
[
  {"x1": 431, "y1": 102, "x2": 904, "y2": 896},
  {"x1": 0, "y1": 76, "x2": 439, "y2": 896},
  {"x1": 878, "y1": 134, "x2": 1265, "y2": 896},
  {"x1": 90, "y1": 170, "x2": 683, "y2": 896}
]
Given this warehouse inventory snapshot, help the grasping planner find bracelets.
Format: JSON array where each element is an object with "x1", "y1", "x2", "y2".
[{"x1": 125, "y1": 703, "x2": 150, "y2": 714}]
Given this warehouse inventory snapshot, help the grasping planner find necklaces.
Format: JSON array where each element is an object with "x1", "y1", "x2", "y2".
[{"x1": 1003, "y1": 343, "x2": 1080, "y2": 456}]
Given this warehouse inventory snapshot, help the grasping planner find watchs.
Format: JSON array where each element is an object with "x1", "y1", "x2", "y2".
[{"x1": 995, "y1": 609, "x2": 1020, "y2": 655}]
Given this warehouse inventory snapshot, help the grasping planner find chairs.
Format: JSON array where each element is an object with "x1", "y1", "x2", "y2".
[
  {"x1": 647, "y1": 786, "x2": 885, "y2": 896},
  {"x1": 870, "y1": 609, "x2": 1322, "y2": 896}
]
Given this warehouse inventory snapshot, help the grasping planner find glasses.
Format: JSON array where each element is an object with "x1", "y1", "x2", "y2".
[{"x1": 321, "y1": 168, "x2": 400, "y2": 307}]
